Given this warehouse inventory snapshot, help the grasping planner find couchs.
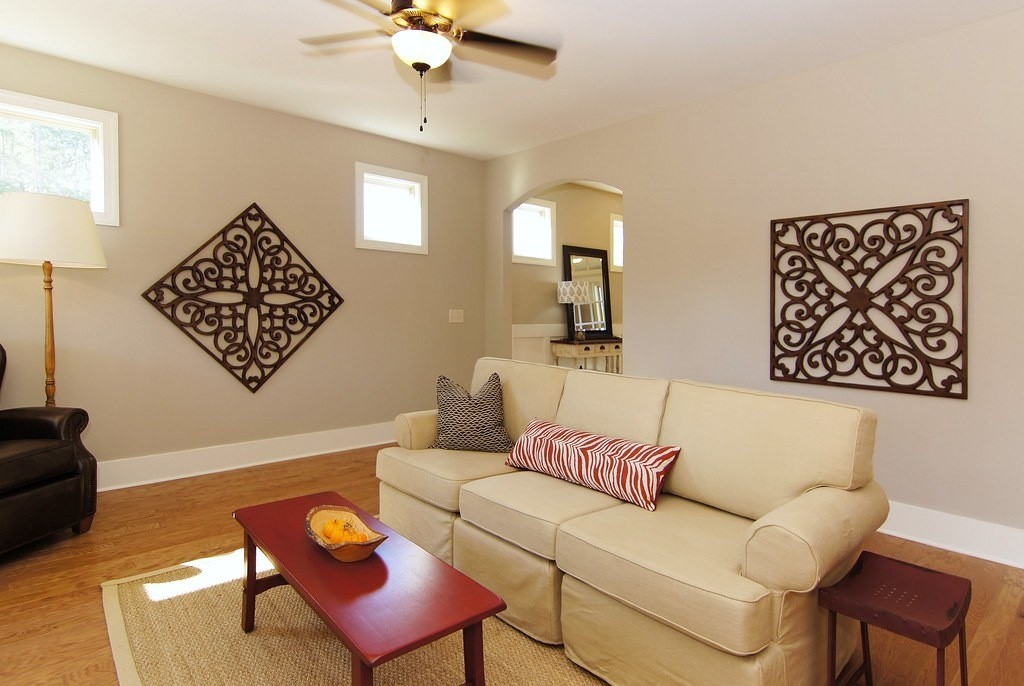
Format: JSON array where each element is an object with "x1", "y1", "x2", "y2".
[
  {"x1": 0, "y1": 344, "x2": 97, "y2": 567},
  {"x1": 376, "y1": 356, "x2": 889, "y2": 686}
]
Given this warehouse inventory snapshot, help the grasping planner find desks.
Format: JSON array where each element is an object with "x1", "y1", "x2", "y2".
[{"x1": 551, "y1": 339, "x2": 623, "y2": 375}]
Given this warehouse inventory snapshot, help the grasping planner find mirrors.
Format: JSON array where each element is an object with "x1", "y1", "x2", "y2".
[{"x1": 562, "y1": 244, "x2": 613, "y2": 340}]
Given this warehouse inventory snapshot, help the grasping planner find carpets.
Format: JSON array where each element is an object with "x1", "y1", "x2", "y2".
[{"x1": 100, "y1": 546, "x2": 612, "y2": 686}]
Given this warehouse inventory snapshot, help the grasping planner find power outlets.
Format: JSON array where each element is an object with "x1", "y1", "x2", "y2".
[{"x1": 449, "y1": 309, "x2": 466, "y2": 323}]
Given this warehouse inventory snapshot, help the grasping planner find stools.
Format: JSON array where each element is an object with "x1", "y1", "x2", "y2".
[{"x1": 817, "y1": 551, "x2": 973, "y2": 686}]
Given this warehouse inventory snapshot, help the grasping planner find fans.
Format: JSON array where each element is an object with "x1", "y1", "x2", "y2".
[{"x1": 299, "y1": 0, "x2": 558, "y2": 67}]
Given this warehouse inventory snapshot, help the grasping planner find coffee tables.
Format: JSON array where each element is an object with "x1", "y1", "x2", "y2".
[{"x1": 233, "y1": 492, "x2": 506, "y2": 686}]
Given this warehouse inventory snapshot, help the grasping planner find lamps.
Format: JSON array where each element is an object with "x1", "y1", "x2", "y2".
[
  {"x1": 0, "y1": 191, "x2": 107, "y2": 407},
  {"x1": 391, "y1": 8, "x2": 452, "y2": 131},
  {"x1": 557, "y1": 281, "x2": 588, "y2": 304}
]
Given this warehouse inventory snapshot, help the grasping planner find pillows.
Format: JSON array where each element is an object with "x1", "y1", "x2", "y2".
[
  {"x1": 506, "y1": 418, "x2": 680, "y2": 512},
  {"x1": 427, "y1": 373, "x2": 515, "y2": 453}
]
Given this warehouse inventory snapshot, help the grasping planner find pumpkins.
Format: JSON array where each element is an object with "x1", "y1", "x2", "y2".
[{"x1": 324, "y1": 518, "x2": 367, "y2": 543}]
